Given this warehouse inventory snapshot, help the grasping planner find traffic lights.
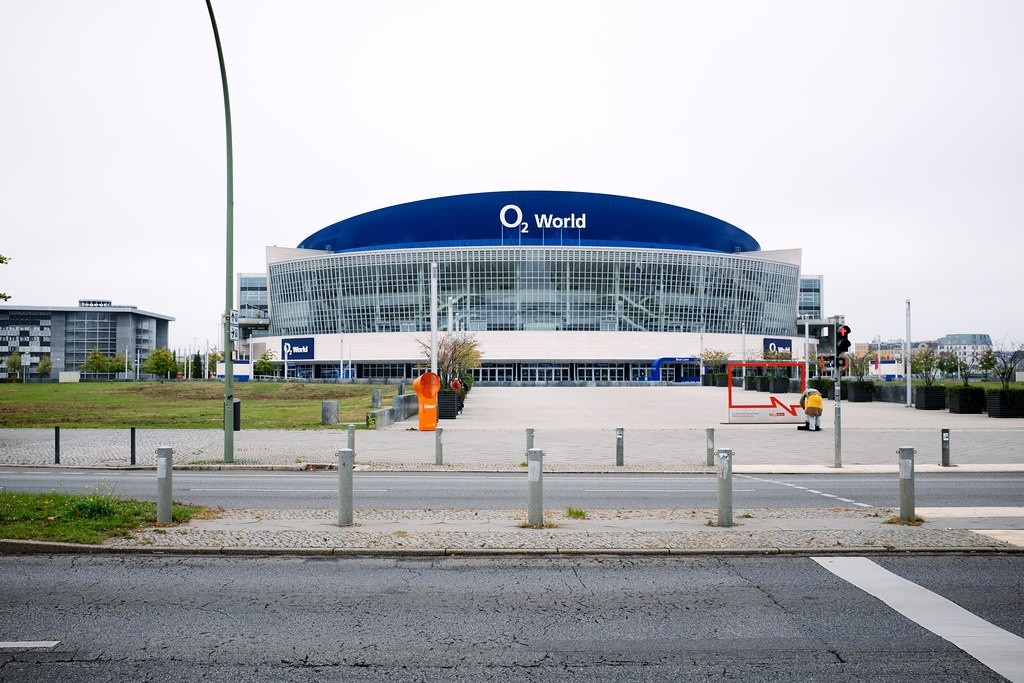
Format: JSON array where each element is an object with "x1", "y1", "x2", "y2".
[
  {"x1": 829, "y1": 356, "x2": 835, "y2": 367},
  {"x1": 836, "y1": 357, "x2": 846, "y2": 367},
  {"x1": 833, "y1": 323, "x2": 851, "y2": 357}
]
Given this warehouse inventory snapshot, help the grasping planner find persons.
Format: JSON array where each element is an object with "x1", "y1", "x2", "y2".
[
  {"x1": 451, "y1": 378, "x2": 463, "y2": 414},
  {"x1": 799, "y1": 388, "x2": 822, "y2": 430}
]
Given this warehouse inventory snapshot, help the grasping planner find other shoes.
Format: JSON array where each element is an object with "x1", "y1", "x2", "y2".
[{"x1": 815, "y1": 426, "x2": 820, "y2": 431}]
